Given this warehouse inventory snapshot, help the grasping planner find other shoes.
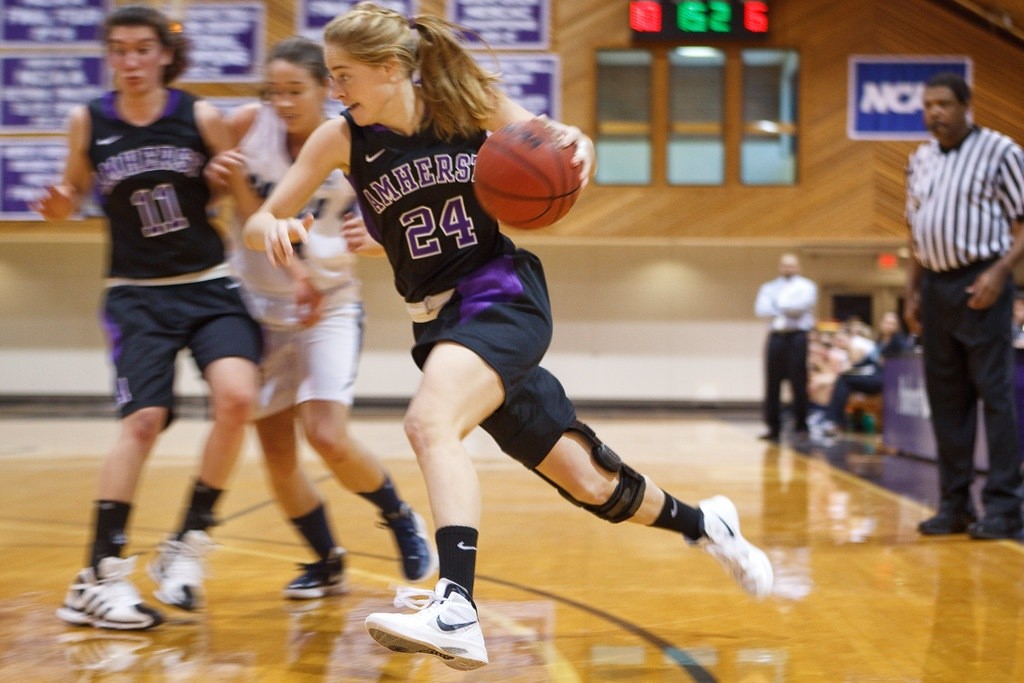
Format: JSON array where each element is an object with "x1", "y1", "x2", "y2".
[
  {"x1": 970, "y1": 513, "x2": 1024, "y2": 539},
  {"x1": 919, "y1": 511, "x2": 976, "y2": 533},
  {"x1": 758, "y1": 431, "x2": 779, "y2": 445}
]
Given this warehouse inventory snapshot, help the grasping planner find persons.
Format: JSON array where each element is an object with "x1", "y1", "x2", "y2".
[
  {"x1": 904, "y1": 73, "x2": 1023, "y2": 539},
  {"x1": 1013, "y1": 297, "x2": 1024, "y2": 349},
  {"x1": 34, "y1": 7, "x2": 322, "y2": 629},
  {"x1": 242, "y1": 0, "x2": 774, "y2": 671},
  {"x1": 806, "y1": 311, "x2": 904, "y2": 435},
  {"x1": 203, "y1": 36, "x2": 436, "y2": 597},
  {"x1": 754, "y1": 253, "x2": 817, "y2": 443}
]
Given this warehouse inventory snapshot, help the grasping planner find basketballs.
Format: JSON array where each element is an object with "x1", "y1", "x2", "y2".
[{"x1": 473, "y1": 117, "x2": 584, "y2": 231}]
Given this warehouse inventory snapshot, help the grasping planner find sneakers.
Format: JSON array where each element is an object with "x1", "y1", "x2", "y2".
[
  {"x1": 364, "y1": 576, "x2": 489, "y2": 670},
  {"x1": 146, "y1": 529, "x2": 215, "y2": 610},
  {"x1": 55, "y1": 555, "x2": 163, "y2": 629},
  {"x1": 283, "y1": 545, "x2": 350, "y2": 598},
  {"x1": 683, "y1": 493, "x2": 771, "y2": 599},
  {"x1": 376, "y1": 502, "x2": 434, "y2": 581}
]
